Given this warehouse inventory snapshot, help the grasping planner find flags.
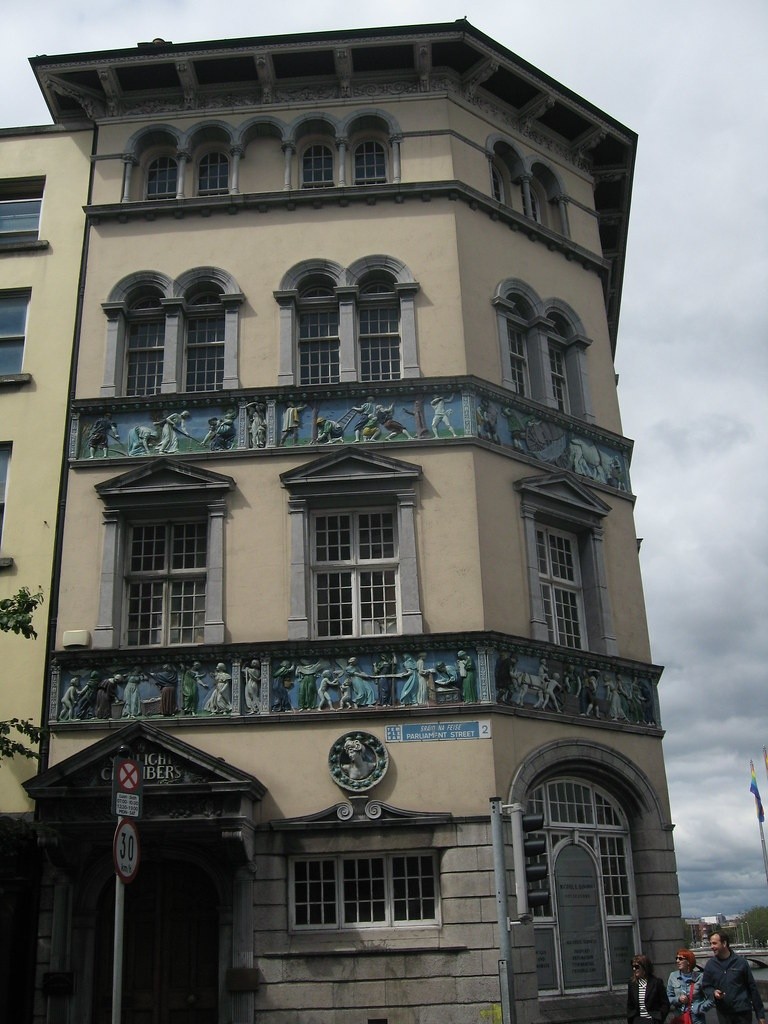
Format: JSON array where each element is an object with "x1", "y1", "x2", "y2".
[{"x1": 748, "y1": 764, "x2": 764, "y2": 823}]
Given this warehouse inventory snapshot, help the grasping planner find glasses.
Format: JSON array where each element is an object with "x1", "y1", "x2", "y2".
[
  {"x1": 676, "y1": 957, "x2": 687, "y2": 960},
  {"x1": 631, "y1": 965, "x2": 641, "y2": 969}
]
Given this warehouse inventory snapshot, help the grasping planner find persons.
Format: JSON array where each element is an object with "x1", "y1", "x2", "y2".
[
  {"x1": 58, "y1": 661, "x2": 231, "y2": 722},
  {"x1": 86, "y1": 403, "x2": 239, "y2": 459},
  {"x1": 702, "y1": 931, "x2": 766, "y2": 1024},
  {"x1": 625, "y1": 954, "x2": 670, "y2": 1024},
  {"x1": 494, "y1": 656, "x2": 658, "y2": 727},
  {"x1": 666, "y1": 949, "x2": 715, "y2": 1024},
  {"x1": 341, "y1": 740, "x2": 376, "y2": 780},
  {"x1": 272, "y1": 648, "x2": 479, "y2": 714},
  {"x1": 241, "y1": 660, "x2": 261, "y2": 714},
  {"x1": 476, "y1": 395, "x2": 628, "y2": 493},
  {"x1": 277, "y1": 391, "x2": 462, "y2": 447},
  {"x1": 252, "y1": 403, "x2": 267, "y2": 448}
]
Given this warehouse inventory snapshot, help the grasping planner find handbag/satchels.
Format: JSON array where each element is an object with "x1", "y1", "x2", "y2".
[{"x1": 670, "y1": 1009, "x2": 692, "y2": 1024}]
